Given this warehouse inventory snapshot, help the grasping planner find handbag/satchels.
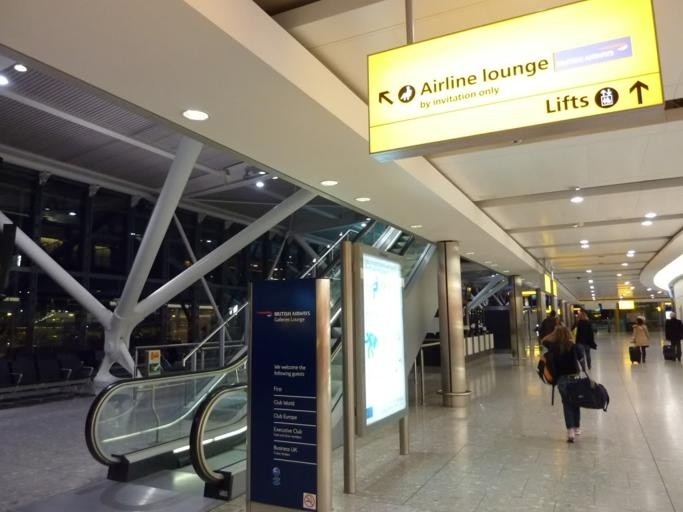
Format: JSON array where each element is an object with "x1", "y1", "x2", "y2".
[
  {"x1": 592, "y1": 342, "x2": 597, "y2": 350},
  {"x1": 537, "y1": 355, "x2": 554, "y2": 386}
]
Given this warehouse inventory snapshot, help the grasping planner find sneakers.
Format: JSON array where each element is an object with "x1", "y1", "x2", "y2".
[{"x1": 567, "y1": 427, "x2": 581, "y2": 442}]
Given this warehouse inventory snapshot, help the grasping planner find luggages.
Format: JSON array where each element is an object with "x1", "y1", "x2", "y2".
[
  {"x1": 663, "y1": 345, "x2": 673, "y2": 360},
  {"x1": 570, "y1": 377, "x2": 609, "y2": 411},
  {"x1": 629, "y1": 341, "x2": 641, "y2": 364}
]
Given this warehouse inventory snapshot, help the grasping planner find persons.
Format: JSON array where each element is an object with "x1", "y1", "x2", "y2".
[
  {"x1": 631, "y1": 317, "x2": 651, "y2": 363},
  {"x1": 539, "y1": 310, "x2": 596, "y2": 443},
  {"x1": 664, "y1": 313, "x2": 681, "y2": 362}
]
